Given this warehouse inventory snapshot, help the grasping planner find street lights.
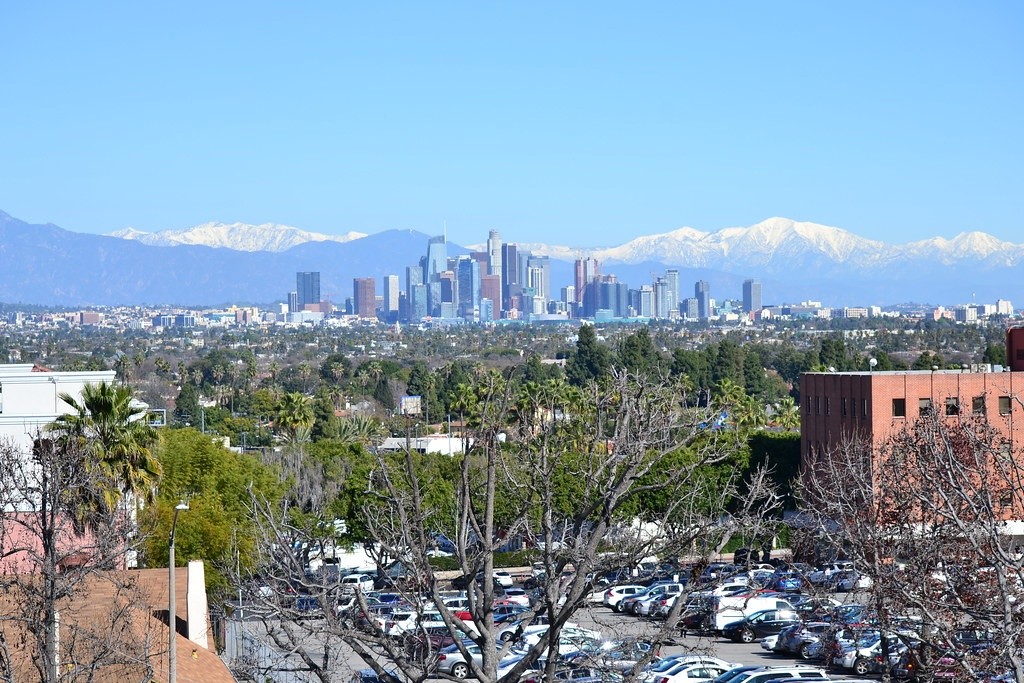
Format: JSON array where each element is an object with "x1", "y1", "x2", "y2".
[{"x1": 167, "y1": 504, "x2": 190, "y2": 683}]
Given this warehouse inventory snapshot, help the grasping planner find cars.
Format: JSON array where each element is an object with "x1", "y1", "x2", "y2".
[{"x1": 250, "y1": 515, "x2": 1024, "y2": 683}]
